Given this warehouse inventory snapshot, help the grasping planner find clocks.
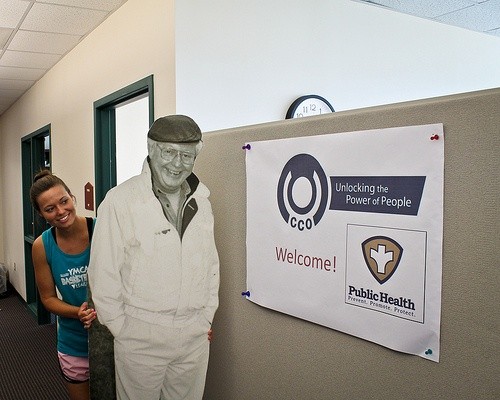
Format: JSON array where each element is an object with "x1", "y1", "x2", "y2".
[{"x1": 285, "y1": 95, "x2": 335, "y2": 119}]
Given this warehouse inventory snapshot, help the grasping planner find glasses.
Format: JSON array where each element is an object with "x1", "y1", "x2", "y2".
[{"x1": 156, "y1": 143, "x2": 197, "y2": 165}]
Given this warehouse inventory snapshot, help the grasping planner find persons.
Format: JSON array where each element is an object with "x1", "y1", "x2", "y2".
[
  {"x1": 30, "y1": 170, "x2": 215, "y2": 400},
  {"x1": 87, "y1": 115, "x2": 219, "y2": 400}
]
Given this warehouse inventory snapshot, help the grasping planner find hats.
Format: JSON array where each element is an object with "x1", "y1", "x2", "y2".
[{"x1": 147, "y1": 115, "x2": 202, "y2": 143}]
